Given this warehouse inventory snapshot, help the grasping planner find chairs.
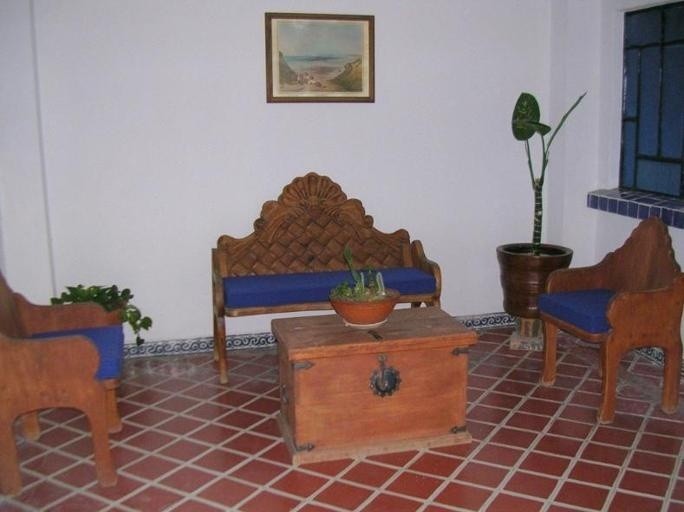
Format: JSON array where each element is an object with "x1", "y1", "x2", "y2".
[
  {"x1": 0, "y1": 272, "x2": 124, "y2": 496},
  {"x1": 534, "y1": 217, "x2": 684, "y2": 428}
]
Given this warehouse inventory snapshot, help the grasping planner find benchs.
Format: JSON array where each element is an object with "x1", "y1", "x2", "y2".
[{"x1": 210, "y1": 172, "x2": 442, "y2": 385}]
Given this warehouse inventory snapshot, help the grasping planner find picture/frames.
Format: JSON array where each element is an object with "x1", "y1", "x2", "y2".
[{"x1": 264, "y1": 12, "x2": 375, "y2": 103}]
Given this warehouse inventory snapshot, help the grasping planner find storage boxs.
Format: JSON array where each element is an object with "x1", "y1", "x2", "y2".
[{"x1": 272, "y1": 309, "x2": 480, "y2": 465}]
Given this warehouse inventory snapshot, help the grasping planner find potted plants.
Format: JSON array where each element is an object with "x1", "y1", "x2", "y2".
[
  {"x1": 496, "y1": 91, "x2": 589, "y2": 319},
  {"x1": 329, "y1": 248, "x2": 403, "y2": 328}
]
